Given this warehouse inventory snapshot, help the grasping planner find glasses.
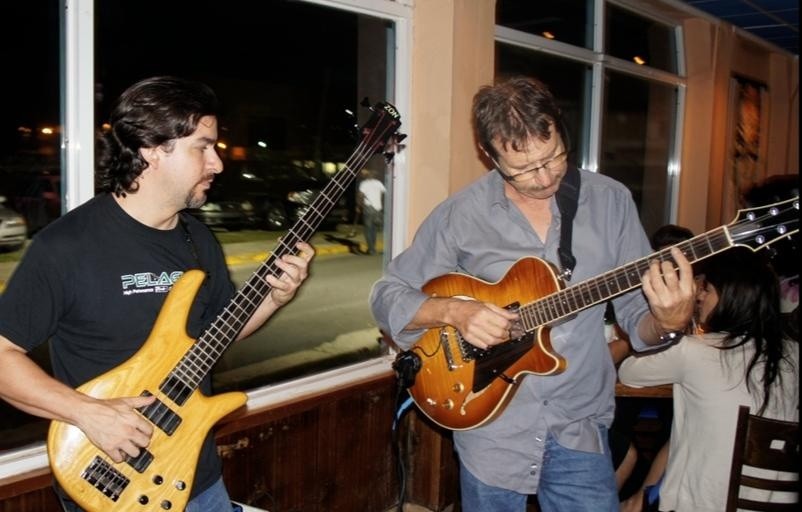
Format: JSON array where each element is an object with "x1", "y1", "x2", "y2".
[{"x1": 488, "y1": 119, "x2": 572, "y2": 183}]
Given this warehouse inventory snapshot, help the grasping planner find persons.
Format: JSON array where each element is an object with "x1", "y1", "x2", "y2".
[
  {"x1": 355, "y1": 179, "x2": 386, "y2": 254},
  {"x1": 370, "y1": 78, "x2": 696, "y2": 512},
  {"x1": 605, "y1": 225, "x2": 696, "y2": 511},
  {"x1": 0, "y1": 76, "x2": 315, "y2": 512},
  {"x1": 618, "y1": 249, "x2": 801, "y2": 510}
]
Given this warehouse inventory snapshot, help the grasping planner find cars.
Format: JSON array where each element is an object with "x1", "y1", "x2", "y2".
[
  {"x1": 0, "y1": 173, "x2": 62, "y2": 249},
  {"x1": 189, "y1": 125, "x2": 352, "y2": 232}
]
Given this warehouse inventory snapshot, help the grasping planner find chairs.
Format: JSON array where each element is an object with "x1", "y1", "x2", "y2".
[{"x1": 724, "y1": 402, "x2": 801, "y2": 512}]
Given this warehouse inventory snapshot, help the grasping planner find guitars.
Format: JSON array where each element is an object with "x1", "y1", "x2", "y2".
[
  {"x1": 397, "y1": 188, "x2": 801, "y2": 431},
  {"x1": 46, "y1": 95, "x2": 407, "y2": 512}
]
